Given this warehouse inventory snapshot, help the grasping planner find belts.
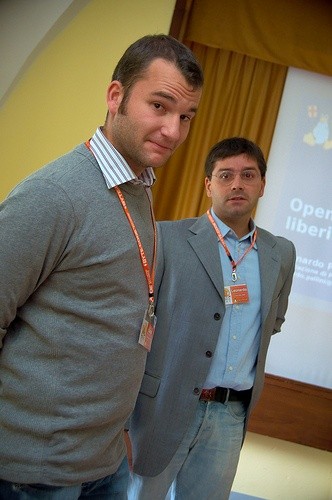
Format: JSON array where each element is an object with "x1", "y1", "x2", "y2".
[{"x1": 199, "y1": 387, "x2": 247, "y2": 403}]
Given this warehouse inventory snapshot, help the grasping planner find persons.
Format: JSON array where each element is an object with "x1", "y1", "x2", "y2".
[
  {"x1": 0, "y1": 34, "x2": 204, "y2": 500},
  {"x1": 125, "y1": 138, "x2": 296, "y2": 500}
]
